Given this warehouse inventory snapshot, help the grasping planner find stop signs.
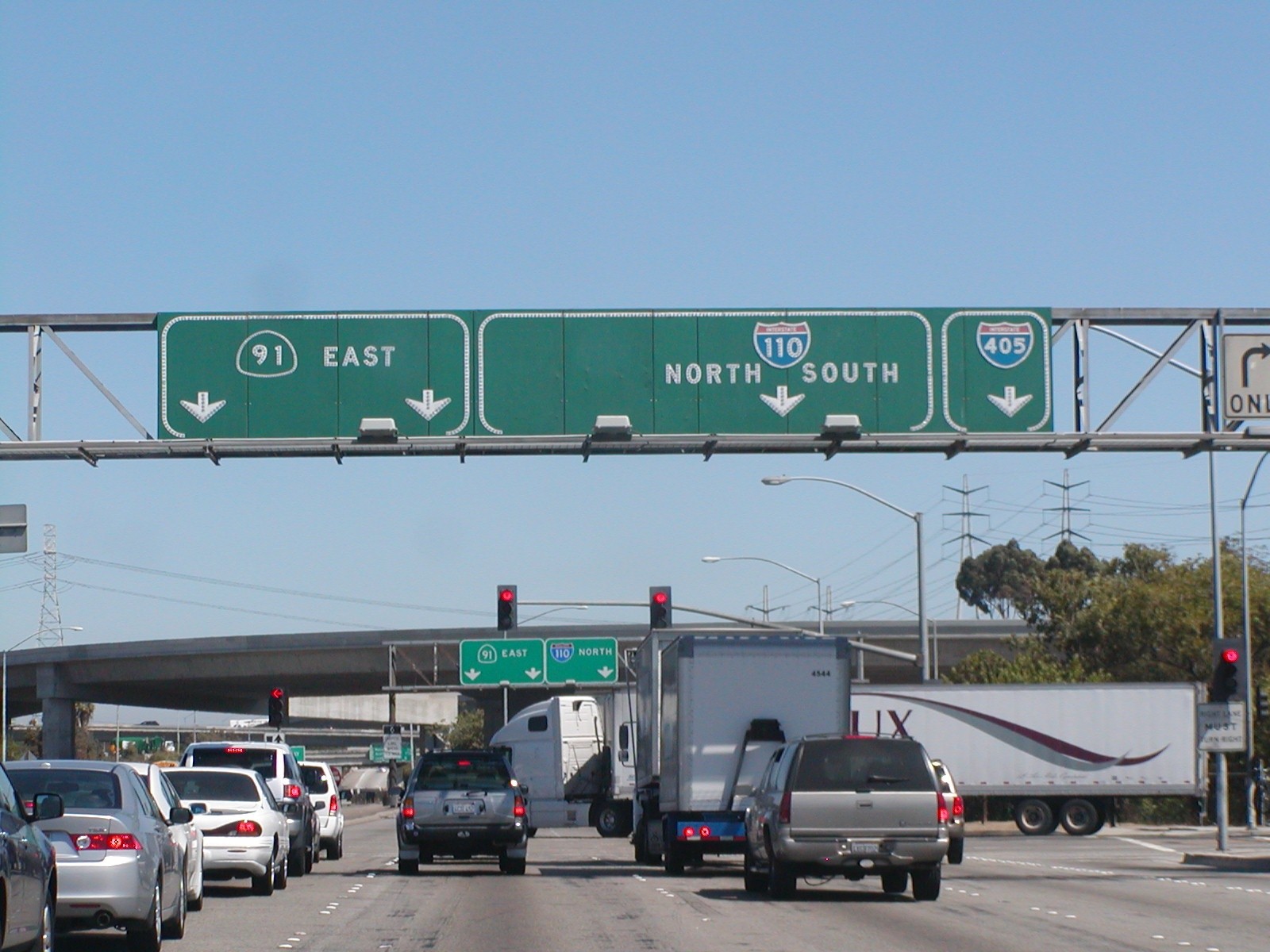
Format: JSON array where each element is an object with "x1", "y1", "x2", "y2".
[{"x1": 330, "y1": 766, "x2": 340, "y2": 784}]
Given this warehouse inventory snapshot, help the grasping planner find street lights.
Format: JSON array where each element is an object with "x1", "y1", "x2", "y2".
[
  {"x1": 837, "y1": 600, "x2": 942, "y2": 682},
  {"x1": 702, "y1": 553, "x2": 826, "y2": 635},
  {"x1": 758, "y1": 473, "x2": 934, "y2": 683},
  {"x1": 0, "y1": 624, "x2": 85, "y2": 763}
]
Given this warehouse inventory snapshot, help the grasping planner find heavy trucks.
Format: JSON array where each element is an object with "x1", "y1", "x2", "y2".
[{"x1": 484, "y1": 678, "x2": 1213, "y2": 836}]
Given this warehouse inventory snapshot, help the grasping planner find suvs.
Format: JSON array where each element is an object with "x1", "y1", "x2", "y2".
[
  {"x1": 394, "y1": 745, "x2": 531, "y2": 878},
  {"x1": 744, "y1": 732, "x2": 968, "y2": 900},
  {"x1": 180, "y1": 741, "x2": 326, "y2": 876}
]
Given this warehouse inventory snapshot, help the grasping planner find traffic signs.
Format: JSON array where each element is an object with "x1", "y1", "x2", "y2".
[
  {"x1": 942, "y1": 305, "x2": 1054, "y2": 438},
  {"x1": 543, "y1": 635, "x2": 619, "y2": 685},
  {"x1": 458, "y1": 637, "x2": 547, "y2": 686},
  {"x1": 1209, "y1": 333, "x2": 1270, "y2": 423},
  {"x1": 155, "y1": 310, "x2": 478, "y2": 442},
  {"x1": 467, "y1": 305, "x2": 944, "y2": 437}
]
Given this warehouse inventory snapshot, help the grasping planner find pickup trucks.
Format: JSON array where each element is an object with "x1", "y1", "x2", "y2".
[{"x1": 632, "y1": 635, "x2": 854, "y2": 877}]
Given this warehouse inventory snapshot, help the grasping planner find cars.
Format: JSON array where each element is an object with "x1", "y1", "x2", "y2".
[
  {"x1": 156, "y1": 765, "x2": 296, "y2": 896},
  {"x1": 114, "y1": 761, "x2": 205, "y2": 934},
  {"x1": 2, "y1": 760, "x2": 194, "y2": 952},
  {"x1": 296, "y1": 759, "x2": 346, "y2": 861},
  {"x1": 0, "y1": 759, "x2": 65, "y2": 952}
]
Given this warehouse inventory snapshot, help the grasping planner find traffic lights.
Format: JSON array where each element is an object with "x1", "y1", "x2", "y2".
[
  {"x1": 496, "y1": 584, "x2": 518, "y2": 634},
  {"x1": 1256, "y1": 685, "x2": 1270, "y2": 725},
  {"x1": 1210, "y1": 638, "x2": 1248, "y2": 703},
  {"x1": 267, "y1": 685, "x2": 290, "y2": 728},
  {"x1": 649, "y1": 585, "x2": 674, "y2": 630}
]
